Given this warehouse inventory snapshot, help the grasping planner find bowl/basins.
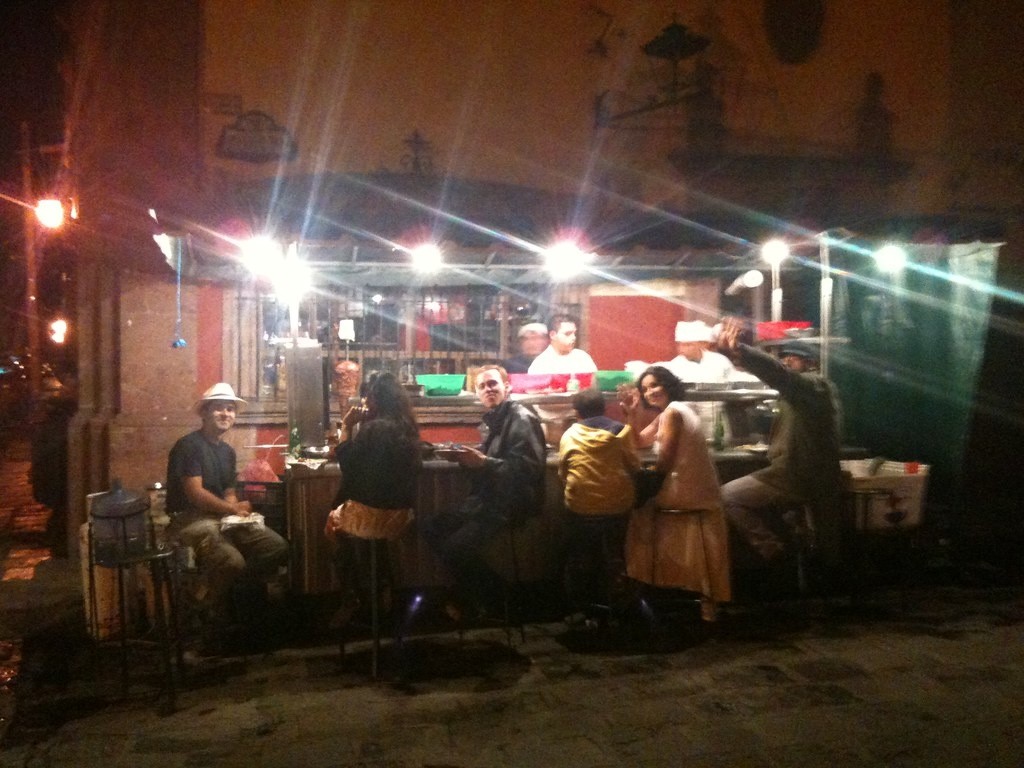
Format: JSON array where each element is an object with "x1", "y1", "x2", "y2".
[
  {"x1": 551, "y1": 373, "x2": 592, "y2": 393},
  {"x1": 415, "y1": 373, "x2": 467, "y2": 396},
  {"x1": 505, "y1": 373, "x2": 552, "y2": 394},
  {"x1": 593, "y1": 370, "x2": 634, "y2": 392}
]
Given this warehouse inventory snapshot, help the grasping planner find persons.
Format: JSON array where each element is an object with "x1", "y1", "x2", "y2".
[
  {"x1": 558, "y1": 388, "x2": 642, "y2": 625},
  {"x1": 528, "y1": 312, "x2": 599, "y2": 374},
  {"x1": 616, "y1": 367, "x2": 728, "y2": 602},
  {"x1": 427, "y1": 365, "x2": 547, "y2": 625},
  {"x1": 675, "y1": 321, "x2": 737, "y2": 441},
  {"x1": 712, "y1": 317, "x2": 840, "y2": 577},
  {"x1": 165, "y1": 382, "x2": 290, "y2": 656},
  {"x1": 325, "y1": 370, "x2": 418, "y2": 627},
  {"x1": 503, "y1": 323, "x2": 548, "y2": 373}
]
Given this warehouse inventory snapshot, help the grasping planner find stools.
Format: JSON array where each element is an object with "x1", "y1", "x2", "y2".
[{"x1": 84, "y1": 488, "x2": 895, "y2": 715}]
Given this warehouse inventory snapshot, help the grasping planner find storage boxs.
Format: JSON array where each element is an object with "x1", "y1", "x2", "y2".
[{"x1": 834, "y1": 457, "x2": 934, "y2": 534}]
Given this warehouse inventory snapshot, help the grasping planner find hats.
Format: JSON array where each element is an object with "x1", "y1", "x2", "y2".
[
  {"x1": 779, "y1": 339, "x2": 812, "y2": 358},
  {"x1": 674, "y1": 320, "x2": 719, "y2": 343},
  {"x1": 195, "y1": 383, "x2": 250, "y2": 414},
  {"x1": 518, "y1": 323, "x2": 549, "y2": 337}
]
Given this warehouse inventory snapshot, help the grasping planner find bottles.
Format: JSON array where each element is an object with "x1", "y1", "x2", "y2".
[
  {"x1": 88, "y1": 477, "x2": 148, "y2": 567},
  {"x1": 566, "y1": 373, "x2": 579, "y2": 394},
  {"x1": 713, "y1": 410, "x2": 725, "y2": 450}
]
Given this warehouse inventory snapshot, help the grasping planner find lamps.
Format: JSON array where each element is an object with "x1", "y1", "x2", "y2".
[{"x1": 589, "y1": 22, "x2": 712, "y2": 129}]
